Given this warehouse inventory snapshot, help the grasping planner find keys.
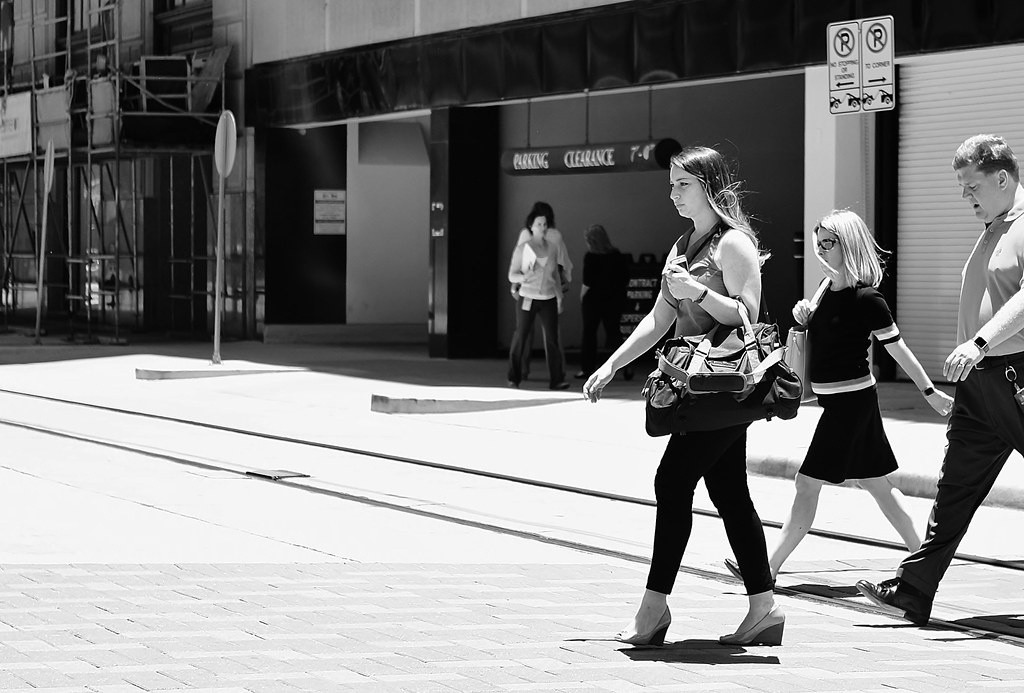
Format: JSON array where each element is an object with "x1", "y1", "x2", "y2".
[{"x1": 1004, "y1": 363, "x2": 1024, "y2": 413}]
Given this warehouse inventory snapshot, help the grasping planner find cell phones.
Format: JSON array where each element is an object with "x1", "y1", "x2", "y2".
[{"x1": 672, "y1": 255, "x2": 691, "y2": 277}]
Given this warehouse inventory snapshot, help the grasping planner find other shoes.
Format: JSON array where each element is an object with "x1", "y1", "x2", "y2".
[
  {"x1": 623, "y1": 360, "x2": 636, "y2": 381},
  {"x1": 521, "y1": 373, "x2": 528, "y2": 381},
  {"x1": 506, "y1": 378, "x2": 518, "y2": 388},
  {"x1": 549, "y1": 382, "x2": 570, "y2": 390},
  {"x1": 573, "y1": 371, "x2": 591, "y2": 380}
]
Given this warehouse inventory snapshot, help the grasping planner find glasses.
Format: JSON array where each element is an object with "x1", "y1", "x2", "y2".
[{"x1": 817, "y1": 237, "x2": 840, "y2": 250}]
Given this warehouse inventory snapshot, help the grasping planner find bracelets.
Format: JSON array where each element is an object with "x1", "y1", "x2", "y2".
[
  {"x1": 691, "y1": 285, "x2": 710, "y2": 305},
  {"x1": 919, "y1": 384, "x2": 937, "y2": 398}
]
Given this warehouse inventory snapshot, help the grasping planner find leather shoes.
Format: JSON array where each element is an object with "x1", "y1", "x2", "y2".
[
  {"x1": 724, "y1": 559, "x2": 776, "y2": 593},
  {"x1": 856, "y1": 580, "x2": 932, "y2": 626}
]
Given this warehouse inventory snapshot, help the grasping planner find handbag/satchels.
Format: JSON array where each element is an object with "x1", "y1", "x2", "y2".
[
  {"x1": 785, "y1": 278, "x2": 831, "y2": 403},
  {"x1": 641, "y1": 301, "x2": 803, "y2": 438}
]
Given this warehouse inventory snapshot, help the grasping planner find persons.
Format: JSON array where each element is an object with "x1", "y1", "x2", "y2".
[
  {"x1": 582, "y1": 146, "x2": 786, "y2": 649},
  {"x1": 724, "y1": 207, "x2": 956, "y2": 593},
  {"x1": 856, "y1": 132, "x2": 1024, "y2": 628},
  {"x1": 504, "y1": 201, "x2": 574, "y2": 390},
  {"x1": 576, "y1": 226, "x2": 634, "y2": 382}
]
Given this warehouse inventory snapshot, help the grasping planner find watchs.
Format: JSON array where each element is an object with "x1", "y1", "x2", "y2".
[{"x1": 971, "y1": 334, "x2": 992, "y2": 361}]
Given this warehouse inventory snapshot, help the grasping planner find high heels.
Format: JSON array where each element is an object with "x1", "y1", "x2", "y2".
[
  {"x1": 720, "y1": 604, "x2": 786, "y2": 647},
  {"x1": 614, "y1": 605, "x2": 671, "y2": 646}
]
